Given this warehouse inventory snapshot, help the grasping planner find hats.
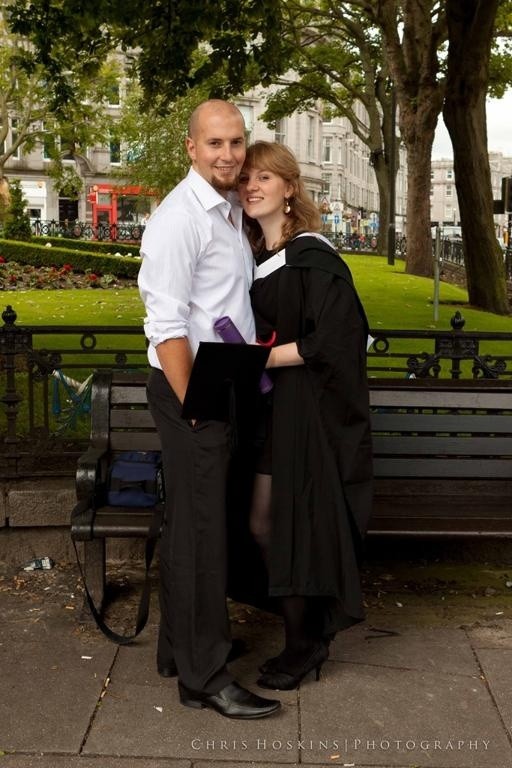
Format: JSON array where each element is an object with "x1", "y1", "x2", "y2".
[{"x1": 180, "y1": 340, "x2": 271, "y2": 419}]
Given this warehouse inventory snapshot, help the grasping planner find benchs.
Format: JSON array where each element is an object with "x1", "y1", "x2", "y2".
[{"x1": 70, "y1": 368, "x2": 512, "y2": 631}]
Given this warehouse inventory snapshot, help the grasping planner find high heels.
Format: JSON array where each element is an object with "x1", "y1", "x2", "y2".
[{"x1": 258, "y1": 643, "x2": 329, "y2": 691}]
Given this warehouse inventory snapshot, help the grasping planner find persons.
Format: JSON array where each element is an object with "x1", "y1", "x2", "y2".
[
  {"x1": 136, "y1": 97, "x2": 283, "y2": 720},
  {"x1": 237, "y1": 139, "x2": 376, "y2": 691},
  {"x1": 140, "y1": 212, "x2": 150, "y2": 225}
]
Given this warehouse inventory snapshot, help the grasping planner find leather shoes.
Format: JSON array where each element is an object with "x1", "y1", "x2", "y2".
[
  {"x1": 157, "y1": 640, "x2": 248, "y2": 678},
  {"x1": 179, "y1": 683, "x2": 282, "y2": 720}
]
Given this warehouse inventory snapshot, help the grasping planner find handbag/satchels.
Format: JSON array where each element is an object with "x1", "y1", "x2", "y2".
[{"x1": 106, "y1": 452, "x2": 163, "y2": 510}]
